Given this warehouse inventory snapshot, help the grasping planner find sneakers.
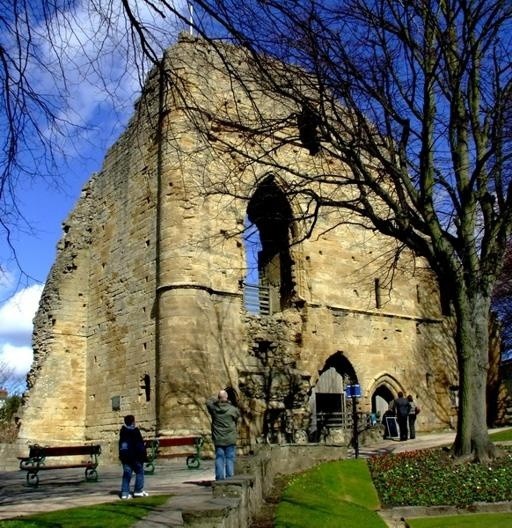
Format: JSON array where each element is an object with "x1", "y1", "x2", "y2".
[{"x1": 122, "y1": 492, "x2": 148, "y2": 499}]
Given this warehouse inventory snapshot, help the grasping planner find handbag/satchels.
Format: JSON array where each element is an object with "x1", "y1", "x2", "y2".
[{"x1": 414, "y1": 407, "x2": 420, "y2": 414}]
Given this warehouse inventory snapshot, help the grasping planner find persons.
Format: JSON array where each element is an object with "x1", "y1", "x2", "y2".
[
  {"x1": 407, "y1": 395, "x2": 416, "y2": 439},
  {"x1": 206, "y1": 390, "x2": 240, "y2": 480},
  {"x1": 119, "y1": 415, "x2": 148, "y2": 499},
  {"x1": 393, "y1": 392, "x2": 411, "y2": 441}
]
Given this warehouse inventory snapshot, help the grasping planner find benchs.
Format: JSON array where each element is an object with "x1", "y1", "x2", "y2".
[
  {"x1": 19, "y1": 441, "x2": 102, "y2": 485},
  {"x1": 141, "y1": 433, "x2": 204, "y2": 475}
]
